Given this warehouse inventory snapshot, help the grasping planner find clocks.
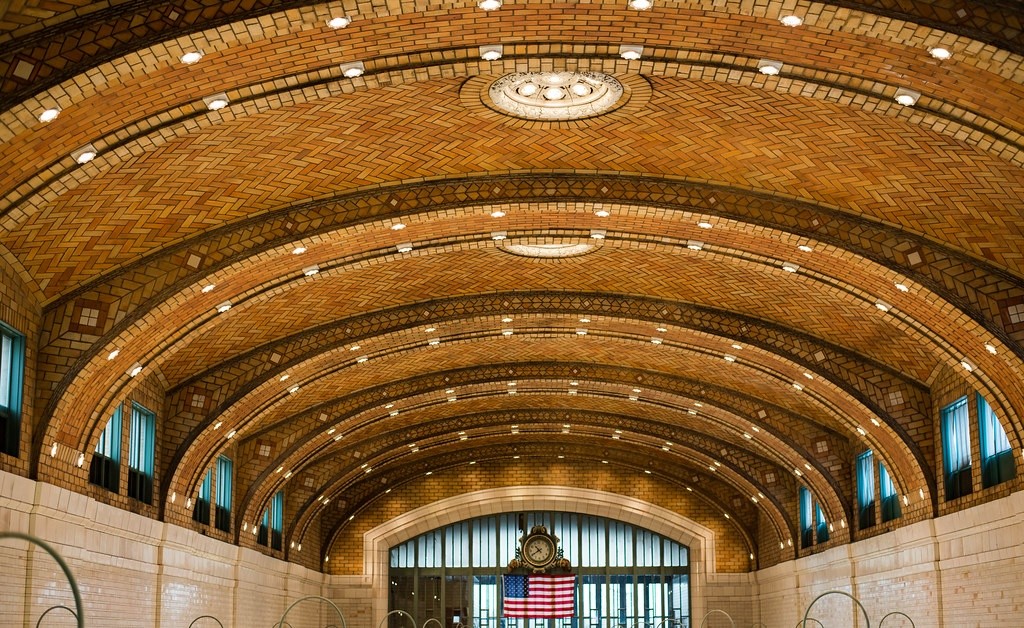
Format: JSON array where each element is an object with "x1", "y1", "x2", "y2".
[{"x1": 526, "y1": 536, "x2": 553, "y2": 564}]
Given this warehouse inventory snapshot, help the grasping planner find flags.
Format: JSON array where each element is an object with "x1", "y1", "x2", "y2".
[{"x1": 502, "y1": 573, "x2": 576, "y2": 619}]
